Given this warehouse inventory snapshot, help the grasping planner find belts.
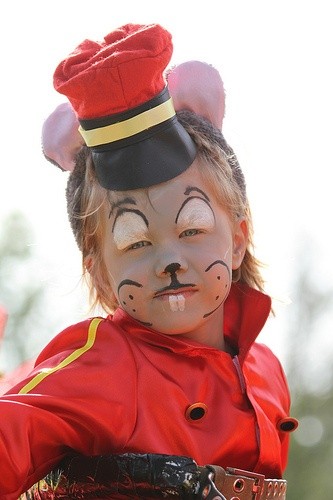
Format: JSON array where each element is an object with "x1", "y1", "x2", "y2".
[{"x1": 18, "y1": 452, "x2": 287, "y2": 500}]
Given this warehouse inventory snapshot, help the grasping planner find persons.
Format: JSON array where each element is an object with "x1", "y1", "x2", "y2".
[{"x1": 1, "y1": 23, "x2": 300, "y2": 500}]
